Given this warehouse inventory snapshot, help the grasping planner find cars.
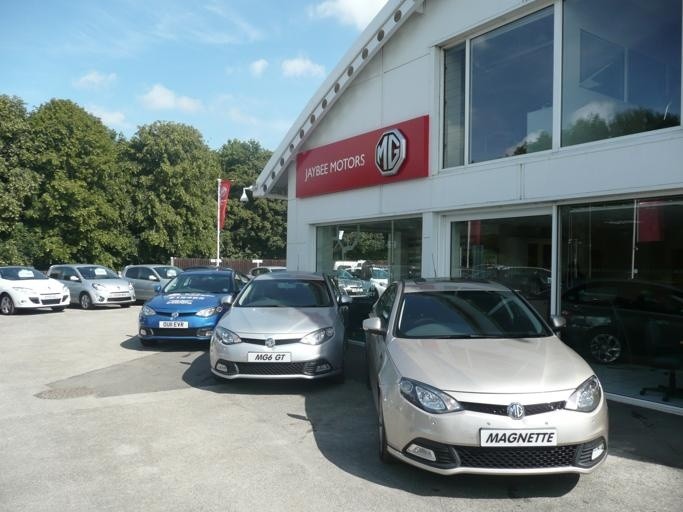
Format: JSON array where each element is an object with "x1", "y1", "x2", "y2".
[
  {"x1": 561, "y1": 280, "x2": 683, "y2": 365},
  {"x1": 209, "y1": 271, "x2": 348, "y2": 387},
  {"x1": 361, "y1": 276, "x2": 609, "y2": 478},
  {"x1": 122, "y1": 264, "x2": 183, "y2": 301},
  {"x1": 138, "y1": 269, "x2": 235, "y2": 344},
  {"x1": 0, "y1": 266, "x2": 71, "y2": 315},
  {"x1": 248, "y1": 259, "x2": 394, "y2": 304}
]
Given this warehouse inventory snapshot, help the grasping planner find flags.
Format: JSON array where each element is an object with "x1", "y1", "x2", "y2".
[
  {"x1": 636, "y1": 197, "x2": 664, "y2": 245},
  {"x1": 218, "y1": 180, "x2": 232, "y2": 230},
  {"x1": 470, "y1": 220, "x2": 482, "y2": 245}
]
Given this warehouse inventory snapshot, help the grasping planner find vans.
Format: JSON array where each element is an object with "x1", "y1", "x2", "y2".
[{"x1": 47, "y1": 264, "x2": 136, "y2": 308}]
